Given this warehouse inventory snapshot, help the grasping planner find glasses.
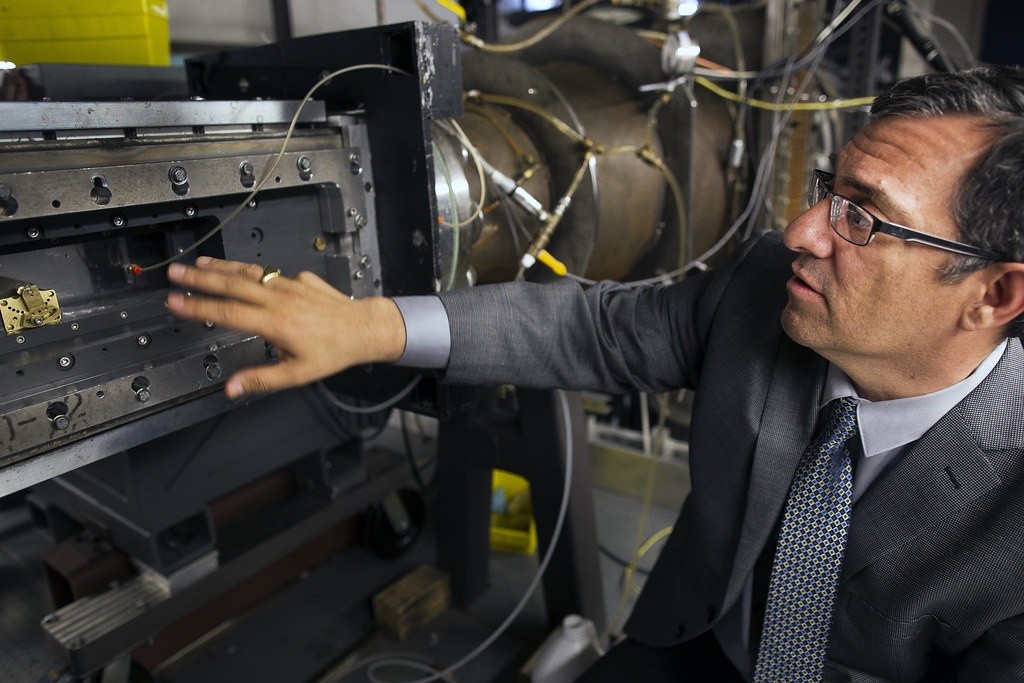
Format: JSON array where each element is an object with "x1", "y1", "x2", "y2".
[{"x1": 808, "y1": 168, "x2": 1013, "y2": 262}]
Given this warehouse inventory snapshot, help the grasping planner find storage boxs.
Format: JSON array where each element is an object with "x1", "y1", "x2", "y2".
[{"x1": 0, "y1": 0, "x2": 180, "y2": 71}]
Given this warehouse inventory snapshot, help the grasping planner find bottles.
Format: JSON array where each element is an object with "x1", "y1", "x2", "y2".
[{"x1": 522, "y1": 614, "x2": 605, "y2": 683}]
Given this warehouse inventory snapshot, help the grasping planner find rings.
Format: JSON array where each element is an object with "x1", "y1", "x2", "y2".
[{"x1": 259, "y1": 264, "x2": 280, "y2": 284}]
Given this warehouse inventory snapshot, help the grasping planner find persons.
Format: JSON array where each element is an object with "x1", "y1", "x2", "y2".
[{"x1": 169, "y1": 65, "x2": 1024, "y2": 683}]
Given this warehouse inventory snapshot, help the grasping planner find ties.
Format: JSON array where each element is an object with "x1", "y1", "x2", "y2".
[{"x1": 753, "y1": 397, "x2": 858, "y2": 683}]
